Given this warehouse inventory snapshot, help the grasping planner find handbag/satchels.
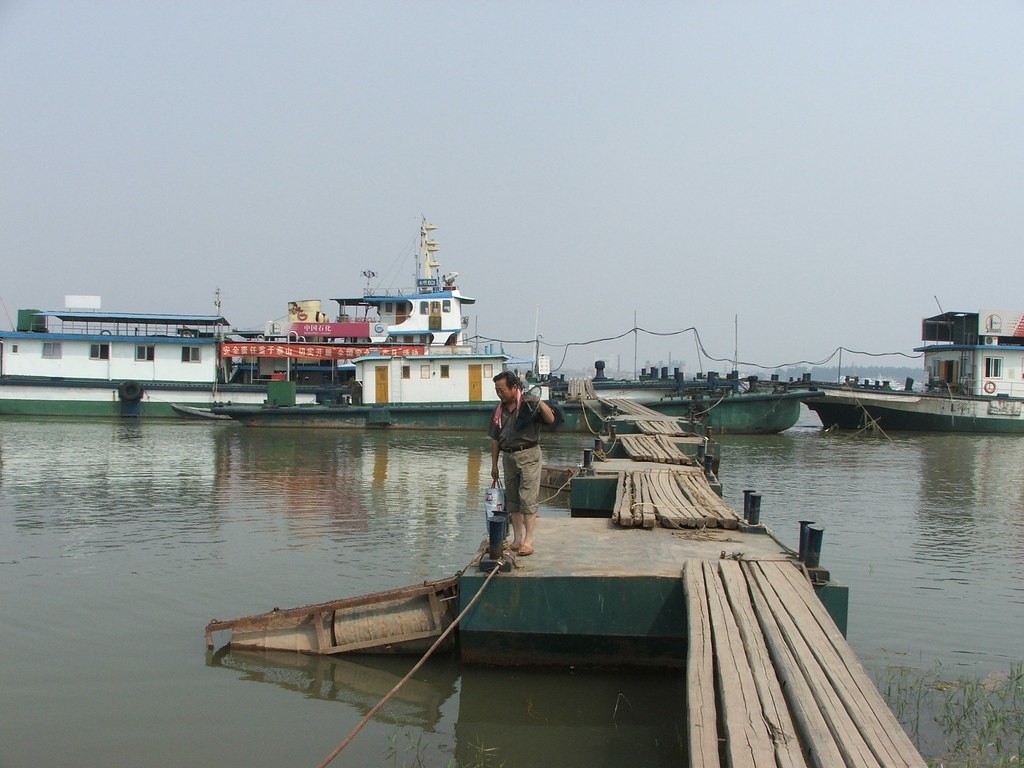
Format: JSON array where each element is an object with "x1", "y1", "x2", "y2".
[{"x1": 484, "y1": 477, "x2": 508, "y2": 539}]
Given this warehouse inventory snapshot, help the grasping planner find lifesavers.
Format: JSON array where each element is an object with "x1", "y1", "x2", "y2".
[
  {"x1": 120, "y1": 379, "x2": 143, "y2": 402},
  {"x1": 984, "y1": 381, "x2": 996, "y2": 394}
]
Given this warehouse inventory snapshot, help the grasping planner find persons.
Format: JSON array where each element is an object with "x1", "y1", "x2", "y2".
[{"x1": 487, "y1": 370, "x2": 558, "y2": 556}]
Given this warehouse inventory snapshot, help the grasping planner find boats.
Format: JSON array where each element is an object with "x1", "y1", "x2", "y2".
[
  {"x1": 169, "y1": 401, "x2": 234, "y2": 420},
  {"x1": 210, "y1": 212, "x2": 825, "y2": 436},
  {"x1": 799, "y1": 311, "x2": 1024, "y2": 438},
  {"x1": 1, "y1": 305, "x2": 356, "y2": 420}
]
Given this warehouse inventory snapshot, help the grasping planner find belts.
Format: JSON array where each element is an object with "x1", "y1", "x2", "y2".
[{"x1": 502, "y1": 441, "x2": 539, "y2": 453}]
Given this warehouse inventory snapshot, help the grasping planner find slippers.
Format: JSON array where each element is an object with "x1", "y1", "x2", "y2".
[{"x1": 509, "y1": 544, "x2": 534, "y2": 556}]
